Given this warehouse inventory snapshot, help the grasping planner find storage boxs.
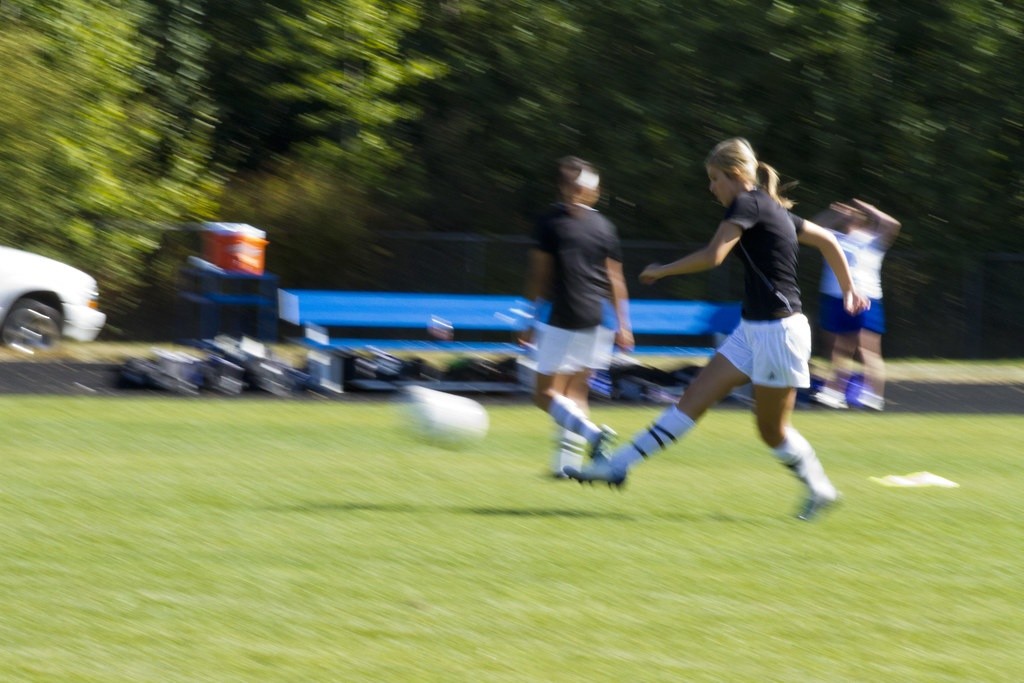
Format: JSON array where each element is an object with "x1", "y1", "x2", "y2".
[{"x1": 202, "y1": 222, "x2": 268, "y2": 275}]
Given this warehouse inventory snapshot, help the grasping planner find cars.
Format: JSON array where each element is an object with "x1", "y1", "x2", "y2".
[{"x1": 0, "y1": 242, "x2": 106, "y2": 352}]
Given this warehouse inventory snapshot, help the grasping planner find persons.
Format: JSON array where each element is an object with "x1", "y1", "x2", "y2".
[
  {"x1": 808, "y1": 197, "x2": 903, "y2": 413},
  {"x1": 562, "y1": 135, "x2": 843, "y2": 522},
  {"x1": 518, "y1": 154, "x2": 636, "y2": 480}
]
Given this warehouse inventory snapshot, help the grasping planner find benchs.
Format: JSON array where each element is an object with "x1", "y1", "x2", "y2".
[{"x1": 279, "y1": 287, "x2": 747, "y2": 393}]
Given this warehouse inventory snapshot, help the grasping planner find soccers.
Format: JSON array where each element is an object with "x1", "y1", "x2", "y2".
[{"x1": 397, "y1": 385, "x2": 490, "y2": 450}]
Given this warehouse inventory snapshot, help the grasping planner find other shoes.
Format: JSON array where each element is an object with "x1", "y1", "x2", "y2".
[
  {"x1": 589, "y1": 425, "x2": 617, "y2": 461},
  {"x1": 793, "y1": 489, "x2": 845, "y2": 523},
  {"x1": 562, "y1": 458, "x2": 627, "y2": 489}
]
